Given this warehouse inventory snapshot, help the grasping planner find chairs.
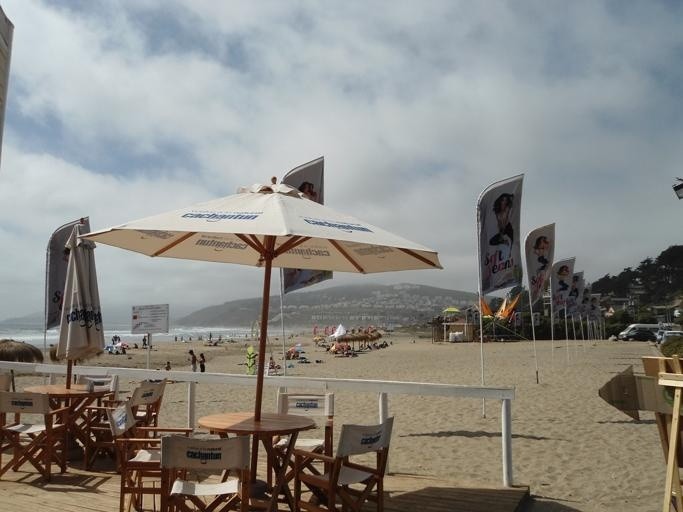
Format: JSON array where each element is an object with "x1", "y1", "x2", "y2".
[
  {"x1": 105, "y1": 388, "x2": 396, "y2": 512},
  {"x1": 0, "y1": 369, "x2": 168, "y2": 482}
]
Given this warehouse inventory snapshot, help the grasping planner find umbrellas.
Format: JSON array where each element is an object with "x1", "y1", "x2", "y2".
[
  {"x1": 54, "y1": 219, "x2": 104, "y2": 458},
  {"x1": 76, "y1": 177, "x2": 445, "y2": 495}
]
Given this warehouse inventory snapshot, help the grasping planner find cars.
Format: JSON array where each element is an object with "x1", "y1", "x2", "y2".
[{"x1": 617, "y1": 321, "x2": 683, "y2": 346}]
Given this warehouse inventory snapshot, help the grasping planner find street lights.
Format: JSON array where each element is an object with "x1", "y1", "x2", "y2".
[{"x1": 671, "y1": 175, "x2": 683, "y2": 199}]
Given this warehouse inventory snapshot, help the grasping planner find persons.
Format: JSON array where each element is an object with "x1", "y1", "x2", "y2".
[
  {"x1": 104, "y1": 324, "x2": 388, "y2": 376},
  {"x1": 581, "y1": 289, "x2": 588, "y2": 304},
  {"x1": 590, "y1": 296, "x2": 596, "y2": 310},
  {"x1": 568, "y1": 276, "x2": 578, "y2": 301},
  {"x1": 533, "y1": 237, "x2": 548, "y2": 280},
  {"x1": 486, "y1": 191, "x2": 514, "y2": 256},
  {"x1": 556, "y1": 264, "x2": 568, "y2": 293}
]
